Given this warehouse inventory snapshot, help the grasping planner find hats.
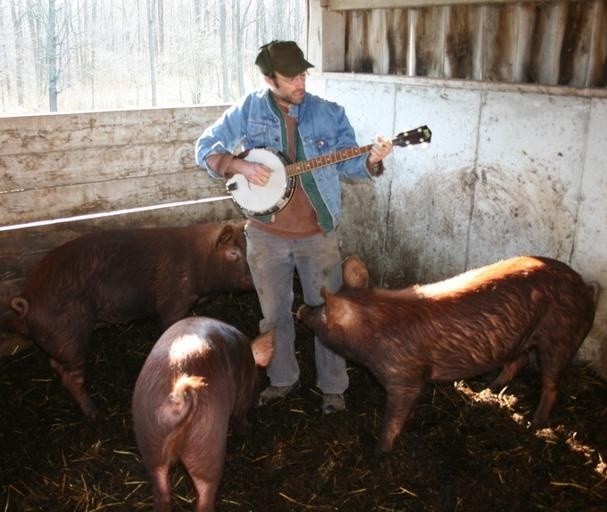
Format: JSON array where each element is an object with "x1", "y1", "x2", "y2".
[{"x1": 255, "y1": 40, "x2": 315, "y2": 77}]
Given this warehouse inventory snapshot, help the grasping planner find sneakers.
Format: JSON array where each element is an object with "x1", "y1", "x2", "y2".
[
  {"x1": 320, "y1": 392, "x2": 346, "y2": 420},
  {"x1": 257, "y1": 379, "x2": 302, "y2": 407}
]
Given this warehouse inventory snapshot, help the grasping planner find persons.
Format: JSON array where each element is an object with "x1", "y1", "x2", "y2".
[{"x1": 193, "y1": 40, "x2": 395, "y2": 414}]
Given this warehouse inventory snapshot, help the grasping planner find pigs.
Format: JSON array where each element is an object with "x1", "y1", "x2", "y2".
[
  {"x1": 130, "y1": 310, "x2": 276, "y2": 509},
  {"x1": 9, "y1": 221, "x2": 256, "y2": 421},
  {"x1": 293, "y1": 253, "x2": 603, "y2": 454}
]
